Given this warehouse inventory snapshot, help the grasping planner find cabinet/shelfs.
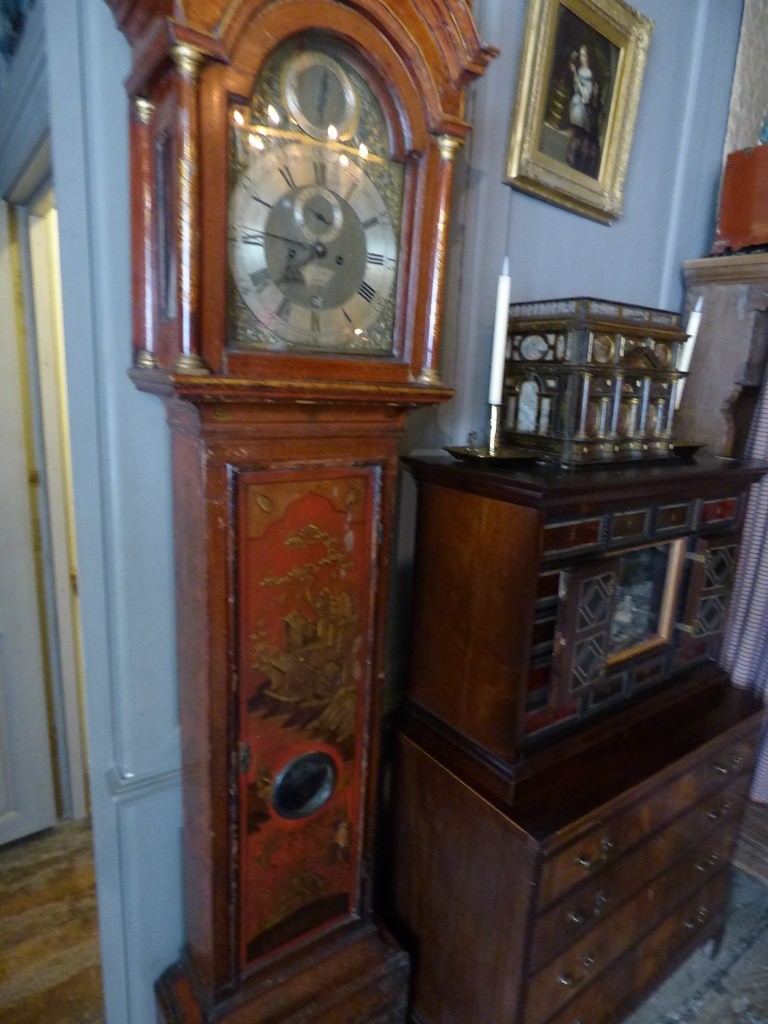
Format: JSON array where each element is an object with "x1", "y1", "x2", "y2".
[
  {"x1": 380, "y1": 686, "x2": 768, "y2": 1024},
  {"x1": 405, "y1": 452, "x2": 768, "y2": 803}
]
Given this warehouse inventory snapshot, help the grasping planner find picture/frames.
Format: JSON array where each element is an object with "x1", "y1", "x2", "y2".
[{"x1": 504, "y1": 0, "x2": 654, "y2": 226}]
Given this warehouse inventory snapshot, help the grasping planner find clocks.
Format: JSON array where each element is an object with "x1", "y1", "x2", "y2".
[{"x1": 221, "y1": 34, "x2": 407, "y2": 357}]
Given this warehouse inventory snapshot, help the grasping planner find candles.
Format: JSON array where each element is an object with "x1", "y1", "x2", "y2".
[
  {"x1": 488, "y1": 256, "x2": 510, "y2": 404},
  {"x1": 676, "y1": 296, "x2": 703, "y2": 411}
]
